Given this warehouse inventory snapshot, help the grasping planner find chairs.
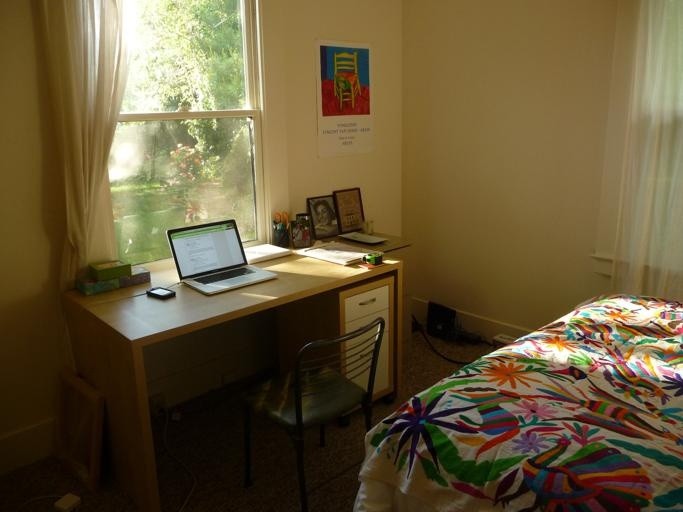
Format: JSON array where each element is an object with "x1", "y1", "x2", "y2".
[
  {"x1": 334, "y1": 52, "x2": 361, "y2": 110},
  {"x1": 238, "y1": 317, "x2": 386, "y2": 512}
]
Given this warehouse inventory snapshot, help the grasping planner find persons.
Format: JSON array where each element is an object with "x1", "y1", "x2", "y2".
[{"x1": 312, "y1": 200, "x2": 337, "y2": 233}]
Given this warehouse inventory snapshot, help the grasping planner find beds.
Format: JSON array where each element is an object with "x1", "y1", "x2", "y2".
[{"x1": 366, "y1": 293, "x2": 683, "y2": 512}]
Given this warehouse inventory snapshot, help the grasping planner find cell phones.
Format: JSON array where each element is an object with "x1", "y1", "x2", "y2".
[{"x1": 146, "y1": 286, "x2": 177, "y2": 299}]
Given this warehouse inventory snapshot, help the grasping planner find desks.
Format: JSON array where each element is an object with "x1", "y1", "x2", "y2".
[{"x1": 63, "y1": 239, "x2": 404, "y2": 512}]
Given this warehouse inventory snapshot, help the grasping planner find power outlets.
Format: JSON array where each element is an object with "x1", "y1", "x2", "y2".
[{"x1": 149, "y1": 392, "x2": 167, "y2": 418}]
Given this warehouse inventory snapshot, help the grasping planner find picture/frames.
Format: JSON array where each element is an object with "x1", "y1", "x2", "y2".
[
  {"x1": 52, "y1": 365, "x2": 104, "y2": 495},
  {"x1": 333, "y1": 188, "x2": 364, "y2": 234},
  {"x1": 307, "y1": 195, "x2": 342, "y2": 240}
]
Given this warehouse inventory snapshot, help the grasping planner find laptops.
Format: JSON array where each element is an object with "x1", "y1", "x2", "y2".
[{"x1": 165, "y1": 219, "x2": 279, "y2": 296}]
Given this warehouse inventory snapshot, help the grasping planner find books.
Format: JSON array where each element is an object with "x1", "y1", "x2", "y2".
[
  {"x1": 297, "y1": 240, "x2": 384, "y2": 264},
  {"x1": 245, "y1": 243, "x2": 290, "y2": 264}
]
